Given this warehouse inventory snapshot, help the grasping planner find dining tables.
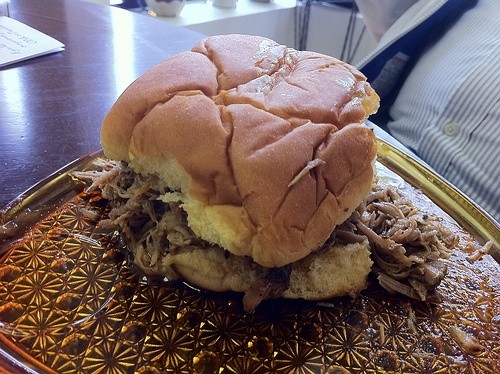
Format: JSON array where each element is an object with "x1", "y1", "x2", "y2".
[{"x1": 0, "y1": 0, "x2": 451, "y2": 279}]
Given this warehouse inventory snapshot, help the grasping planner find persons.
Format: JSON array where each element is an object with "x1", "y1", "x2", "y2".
[{"x1": 354, "y1": 0, "x2": 500, "y2": 226}]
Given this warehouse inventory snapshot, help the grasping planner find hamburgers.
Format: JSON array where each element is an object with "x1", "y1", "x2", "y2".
[{"x1": 67, "y1": 33, "x2": 459, "y2": 315}]
